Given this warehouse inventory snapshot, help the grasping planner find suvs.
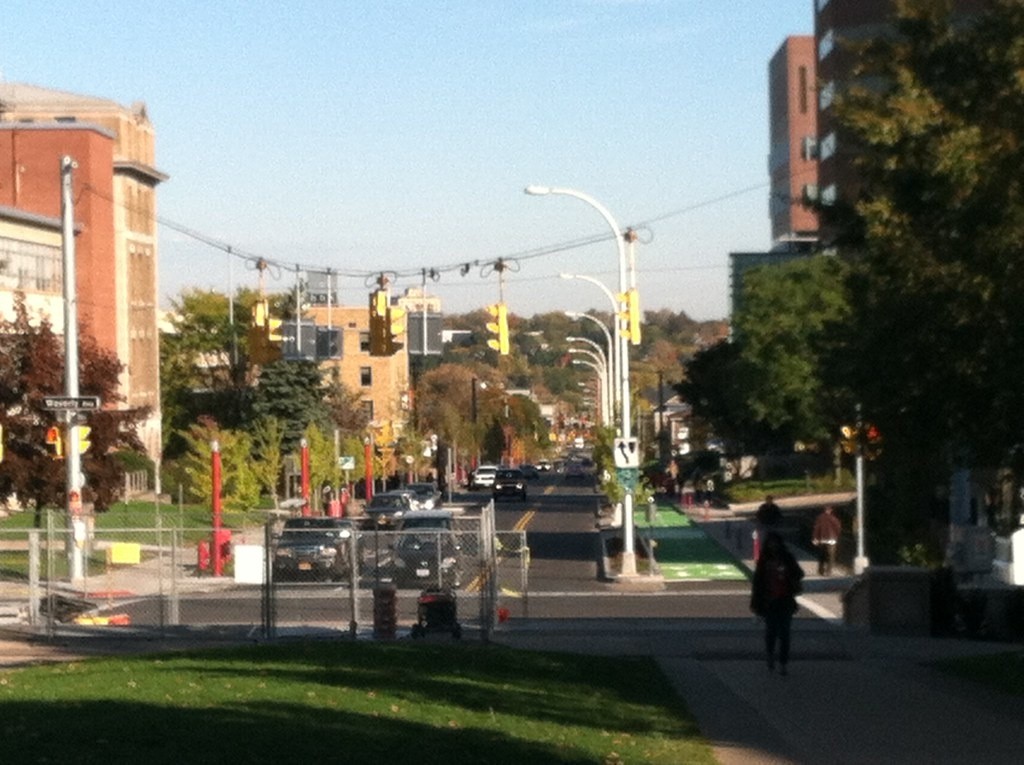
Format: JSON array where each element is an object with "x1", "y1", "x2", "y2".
[
  {"x1": 491, "y1": 466, "x2": 527, "y2": 501},
  {"x1": 273, "y1": 517, "x2": 364, "y2": 584},
  {"x1": 389, "y1": 511, "x2": 465, "y2": 589}
]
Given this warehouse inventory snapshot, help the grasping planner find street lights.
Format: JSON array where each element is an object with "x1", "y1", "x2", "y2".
[
  {"x1": 557, "y1": 272, "x2": 625, "y2": 534},
  {"x1": 525, "y1": 185, "x2": 637, "y2": 581}
]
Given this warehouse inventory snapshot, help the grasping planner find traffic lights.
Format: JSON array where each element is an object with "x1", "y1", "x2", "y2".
[
  {"x1": 387, "y1": 308, "x2": 406, "y2": 340},
  {"x1": 76, "y1": 426, "x2": 94, "y2": 457},
  {"x1": 44, "y1": 428, "x2": 63, "y2": 458},
  {"x1": 614, "y1": 288, "x2": 641, "y2": 346},
  {"x1": 265, "y1": 317, "x2": 286, "y2": 347},
  {"x1": 483, "y1": 304, "x2": 510, "y2": 357},
  {"x1": 840, "y1": 424, "x2": 856, "y2": 455},
  {"x1": 859, "y1": 422, "x2": 884, "y2": 456}
]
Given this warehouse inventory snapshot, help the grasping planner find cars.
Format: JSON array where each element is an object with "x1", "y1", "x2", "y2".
[
  {"x1": 391, "y1": 483, "x2": 445, "y2": 516},
  {"x1": 359, "y1": 494, "x2": 416, "y2": 531},
  {"x1": 522, "y1": 459, "x2": 554, "y2": 482},
  {"x1": 563, "y1": 465, "x2": 583, "y2": 481},
  {"x1": 470, "y1": 464, "x2": 501, "y2": 490}
]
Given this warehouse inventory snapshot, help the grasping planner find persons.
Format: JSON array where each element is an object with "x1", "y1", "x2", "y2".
[
  {"x1": 749, "y1": 532, "x2": 805, "y2": 675},
  {"x1": 663, "y1": 459, "x2": 679, "y2": 496},
  {"x1": 812, "y1": 504, "x2": 842, "y2": 577},
  {"x1": 754, "y1": 495, "x2": 783, "y2": 530}
]
